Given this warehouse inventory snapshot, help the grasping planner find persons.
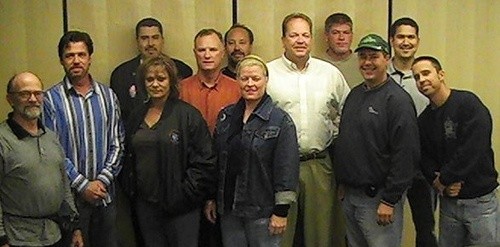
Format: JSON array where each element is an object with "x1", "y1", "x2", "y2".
[
  {"x1": 204, "y1": 54, "x2": 302, "y2": 247},
  {"x1": 385, "y1": 17, "x2": 439, "y2": 247},
  {"x1": 109, "y1": 17, "x2": 194, "y2": 129},
  {"x1": 122, "y1": 58, "x2": 220, "y2": 247},
  {"x1": 221, "y1": 21, "x2": 255, "y2": 80},
  {"x1": 175, "y1": 28, "x2": 243, "y2": 140},
  {"x1": 334, "y1": 36, "x2": 421, "y2": 247},
  {"x1": 312, "y1": 13, "x2": 367, "y2": 246},
  {"x1": 44, "y1": 30, "x2": 128, "y2": 247},
  {"x1": 0, "y1": 71, "x2": 83, "y2": 247},
  {"x1": 410, "y1": 55, "x2": 500, "y2": 247},
  {"x1": 263, "y1": 12, "x2": 352, "y2": 247}
]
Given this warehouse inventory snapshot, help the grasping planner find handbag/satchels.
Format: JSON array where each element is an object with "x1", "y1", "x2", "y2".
[{"x1": 53, "y1": 199, "x2": 75, "y2": 243}]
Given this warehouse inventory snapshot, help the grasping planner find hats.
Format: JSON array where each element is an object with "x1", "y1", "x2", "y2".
[{"x1": 354, "y1": 34, "x2": 389, "y2": 53}]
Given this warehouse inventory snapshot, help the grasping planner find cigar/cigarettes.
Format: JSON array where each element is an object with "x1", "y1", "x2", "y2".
[{"x1": 102, "y1": 198, "x2": 108, "y2": 209}]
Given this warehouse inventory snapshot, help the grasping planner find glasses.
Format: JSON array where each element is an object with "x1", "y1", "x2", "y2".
[{"x1": 8, "y1": 91, "x2": 44, "y2": 98}]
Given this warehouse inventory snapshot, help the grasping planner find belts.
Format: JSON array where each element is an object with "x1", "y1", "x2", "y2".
[{"x1": 300, "y1": 150, "x2": 326, "y2": 162}]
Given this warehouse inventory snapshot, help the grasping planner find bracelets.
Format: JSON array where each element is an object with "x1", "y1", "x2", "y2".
[{"x1": 205, "y1": 200, "x2": 215, "y2": 206}]
